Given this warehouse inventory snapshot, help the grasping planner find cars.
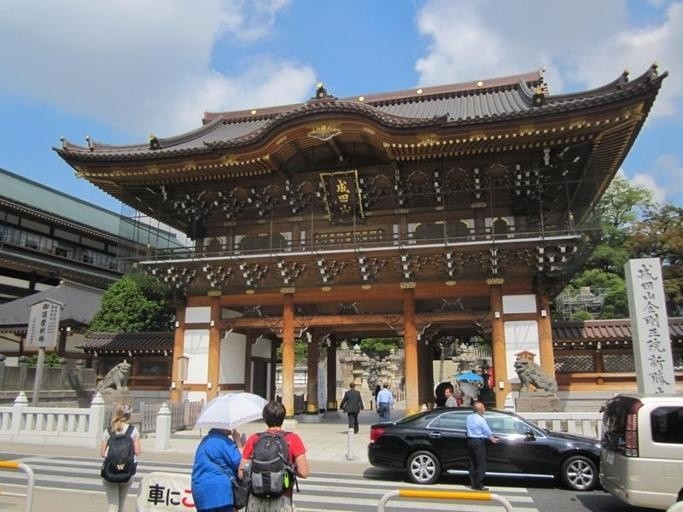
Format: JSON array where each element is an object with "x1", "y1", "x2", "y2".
[{"x1": 368, "y1": 407, "x2": 601, "y2": 490}]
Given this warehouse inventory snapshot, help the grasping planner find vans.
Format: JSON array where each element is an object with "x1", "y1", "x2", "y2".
[{"x1": 599, "y1": 393, "x2": 683, "y2": 510}]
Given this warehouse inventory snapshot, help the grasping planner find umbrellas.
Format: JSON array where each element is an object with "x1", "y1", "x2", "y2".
[
  {"x1": 194, "y1": 392, "x2": 269, "y2": 433},
  {"x1": 453, "y1": 372, "x2": 484, "y2": 401}
]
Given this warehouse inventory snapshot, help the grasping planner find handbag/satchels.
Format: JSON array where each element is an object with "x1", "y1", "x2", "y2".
[{"x1": 232, "y1": 469, "x2": 249, "y2": 509}]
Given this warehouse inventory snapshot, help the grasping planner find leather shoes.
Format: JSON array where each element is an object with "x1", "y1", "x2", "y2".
[{"x1": 471, "y1": 486, "x2": 489, "y2": 491}]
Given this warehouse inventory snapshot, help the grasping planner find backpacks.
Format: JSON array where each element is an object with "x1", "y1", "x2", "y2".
[
  {"x1": 101, "y1": 424, "x2": 138, "y2": 483},
  {"x1": 249, "y1": 432, "x2": 293, "y2": 499}
]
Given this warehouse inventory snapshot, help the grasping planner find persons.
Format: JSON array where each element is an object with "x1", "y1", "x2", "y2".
[
  {"x1": 191, "y1": 428, "x2": 243, "y2": 512},
  {"x1": 377, "y1": 383, "x2": 394, "y2": 422},
  {"x1": 238, "y1": 400, "x2": 309, "y2": 512},
  {"x1": 466, "y1": 402, "x2": 497, "y2": 491},
  {"x1": 444, "y1": 387, "x2": 457, "y2": 407},
  {"x1": 459, "y1": 393, "x2": 472, "y2": 407},
  {"x1": 340, "y1": 382, "x2": 364, "y2": 434},
  {"x1": 375, "y1": 385, "x2": 381, "y2": 413},
  {"x1": 100, "y1": 403, "x2": 140, "y2": 512}
]
